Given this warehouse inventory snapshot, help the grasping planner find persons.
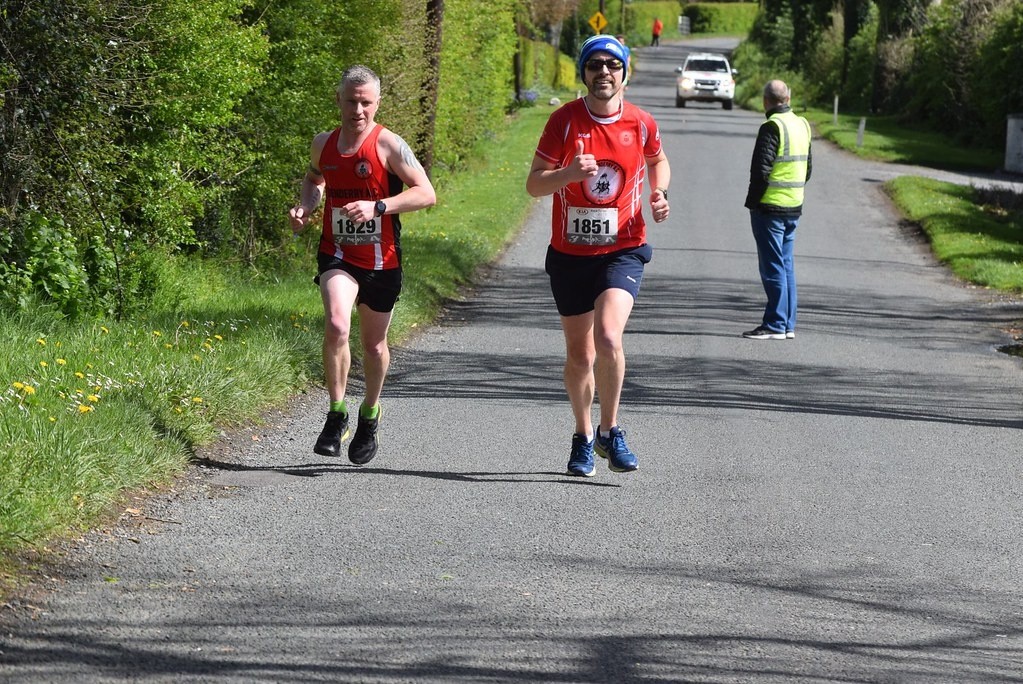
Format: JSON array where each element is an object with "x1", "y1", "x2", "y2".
[
  {"x1": 744, "y1": 79, "x2": 812, "y2": 339},
  {"x1": 289, "y1": 65, "x2": 436, "y2": 463},
  {"x1": 526, "y1": 33, "x2": 670, "y2": 476},
  {"x1": 650, "y1": 18, "x2": 662, "y2": 47},
  {"x1": 617, "y1": 35, "x2": 632, "y2": 91}
]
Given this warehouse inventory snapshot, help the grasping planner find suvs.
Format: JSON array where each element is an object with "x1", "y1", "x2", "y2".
[{"x1": 674, "y1": 51, "x2": 739, "y2": 110}]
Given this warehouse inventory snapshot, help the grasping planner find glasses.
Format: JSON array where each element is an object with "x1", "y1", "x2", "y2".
[{"x1": 585, "y1": 58, "x2": 624, "y2": 70}]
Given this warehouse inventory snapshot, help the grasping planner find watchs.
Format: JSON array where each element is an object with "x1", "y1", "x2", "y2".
[
  {"x1": 657, "y1": 187, "x2": 668, "y2": 200},
  {"x1": 375, "y1": 200, "x2": 386, "y2": 217}
]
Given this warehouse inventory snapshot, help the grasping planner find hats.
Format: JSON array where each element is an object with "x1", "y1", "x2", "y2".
[{"x1": 579, "y1": 34, "x2": 628, "y2": 86}]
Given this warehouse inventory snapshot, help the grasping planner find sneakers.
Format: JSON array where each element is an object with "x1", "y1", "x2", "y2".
[
  {"x1": 348, "y1": 402, "x2": 381, "y2": 464},
  {"x1": 742, "y1": 326, "x2": 786, "y2": 340},
  {"x1": 786, "y1": 331, "x2": 795, "y2": 338},
  {"x1": 593, "y1": 425, "x2": 639, "y2": 472},
  {"x1": 314, "y1": 412, "x2": 350, "y2": 456},
  {"x1": 567, "y1": 430, "x2": 596, "y2": 477}
]
